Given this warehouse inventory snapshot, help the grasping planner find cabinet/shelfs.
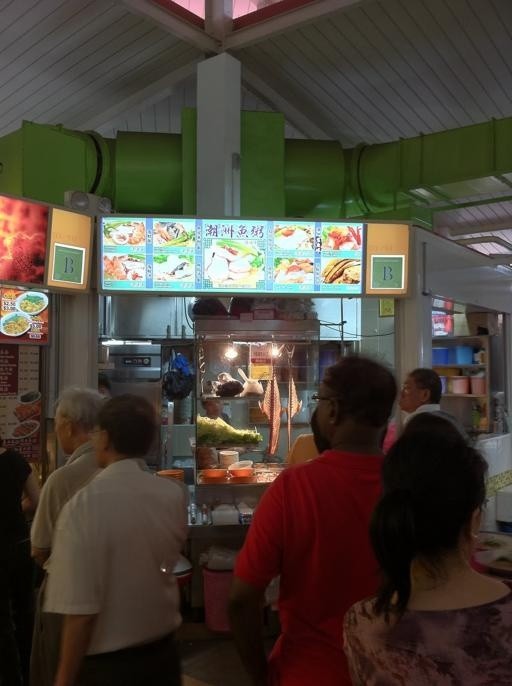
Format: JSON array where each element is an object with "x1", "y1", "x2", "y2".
[
  {"x1": 432, "y1": 334, "x2": 495, "y2": 438},
  {"x1": 183, "y1": 463, "x2": 290, "y2": 621}
]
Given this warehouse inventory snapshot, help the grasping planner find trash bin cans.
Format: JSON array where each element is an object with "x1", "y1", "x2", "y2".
[
  {"x1": 173, "y1": 552, "x2": 193, "y2": 623},
  {"x1": 201, "y1": 545, "x2": 236, "y2": 631}
]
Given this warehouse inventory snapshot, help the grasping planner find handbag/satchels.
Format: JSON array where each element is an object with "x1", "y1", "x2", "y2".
[{"x1": 163, "y1": 345, "x2": 193, "y2": 402}]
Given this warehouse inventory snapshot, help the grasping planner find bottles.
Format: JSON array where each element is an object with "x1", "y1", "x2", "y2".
[
  {"x1": 191, "y1": 502, "x2": 197, "y2": 524},
  {"x1": 201, "y1": 504, "x2": 209, "y2": 525}
]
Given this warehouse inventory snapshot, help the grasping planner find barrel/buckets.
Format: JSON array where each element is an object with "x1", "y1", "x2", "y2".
[{"x1": 202, "y1": 568, "x2": 234, "y2": 632}]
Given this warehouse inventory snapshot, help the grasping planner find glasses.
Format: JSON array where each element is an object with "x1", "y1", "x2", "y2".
[
  {"x1": 312, "y1": 392, "x2": 330, "y2": 402},
  {"x1": 86, "y1": 430, "x2": 107, "y2": 439}
]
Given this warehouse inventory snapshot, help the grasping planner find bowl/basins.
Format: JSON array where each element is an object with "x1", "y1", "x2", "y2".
[
  {"x1": 201, "y1": 459, "x2": 255, "y2": 479},
  {"x1": 155, "y1": 469, "x2": 184, "y2": 486}
]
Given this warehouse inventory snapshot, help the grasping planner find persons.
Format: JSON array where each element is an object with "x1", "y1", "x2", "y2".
[
  {"x1": 400, "y1": 369, "x2": 442, "y2": 427},
  {"x1": 234, "y1": 358, "x2": 397, "y2": 686},
  {"x1": 196, "y1": 394, "x2": 259, "y2": 469},
  {"x1": 342, "y1": 410, "x2": 512, "y2": 685},
  {"x1": 25, "y1": 383, "x2": 109, "y2": 686},
  {"x1": 310, "y1": 407, "x2": 332, "y2": 457},
  {"x1": 1, "y1": 439, "x2": 43, "y2": 684},
  {"x1": 42, "y1": 389, "x2": 191, "y2": 685}
]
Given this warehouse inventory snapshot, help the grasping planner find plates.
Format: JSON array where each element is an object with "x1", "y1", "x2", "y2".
[
  {"x1": 12, "y1": 390, "x2": 42, "y2": 439},
  {"x1": 0, "y1": 291, "x2": 49, "y2": 336}
]
